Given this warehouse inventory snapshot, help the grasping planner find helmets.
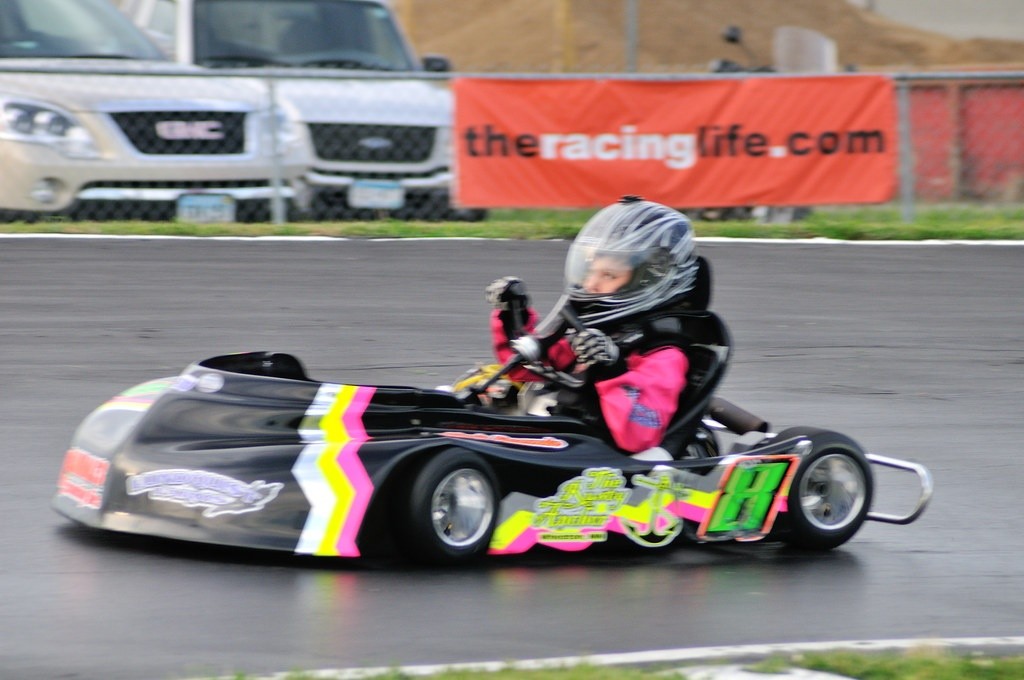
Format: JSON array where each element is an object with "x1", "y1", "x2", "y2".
[{"x1": 564, "y1": 197, "x2": 700, "y2": 326}]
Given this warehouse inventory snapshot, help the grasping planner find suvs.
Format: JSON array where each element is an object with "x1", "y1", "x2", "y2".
[
  {"x1": 120, "y1": 0, "x2": 456, "y2": 221},
  {"x1": 1, "y1": 0, "x2": 313, "y2": 224}
]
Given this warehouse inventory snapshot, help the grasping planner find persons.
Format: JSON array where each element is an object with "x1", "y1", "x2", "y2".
[{"x1": 438, "y1": 197, "x2": 700, "y2": 451}]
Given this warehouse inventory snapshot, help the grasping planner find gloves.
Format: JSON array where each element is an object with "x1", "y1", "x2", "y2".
[
  {"x1": 487, "y1": 275, "x2": 529, "y2": 314},
  {"x1": 566, "y1": 326, "x2": 620, "y2": 367}
]
